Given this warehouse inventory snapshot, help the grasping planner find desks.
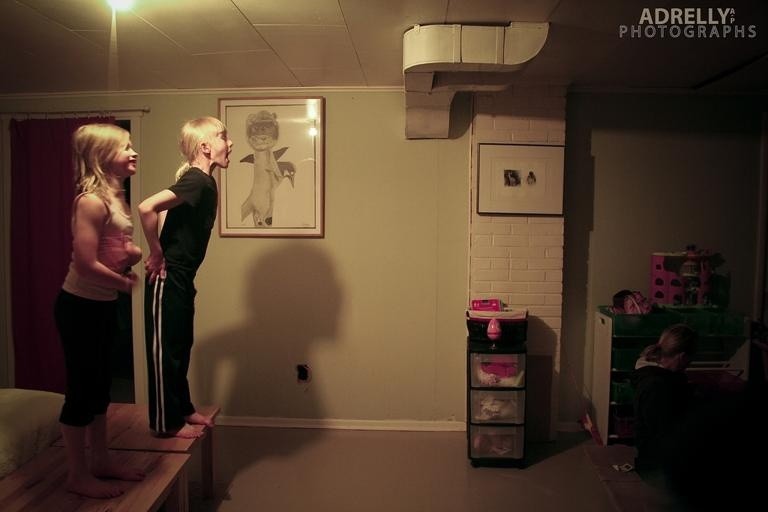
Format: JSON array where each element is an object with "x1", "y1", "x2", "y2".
[{"x1": 4, "y1": 398, "x2": 223, "y2": 512}]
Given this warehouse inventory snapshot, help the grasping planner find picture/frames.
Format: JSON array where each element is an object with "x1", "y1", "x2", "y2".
[
  {"x1": 475, "y1": 138, "x2": 568, "y2": 218},
  {"x1": 215, "y1": 95, "x2": 324, "y2": 237}
]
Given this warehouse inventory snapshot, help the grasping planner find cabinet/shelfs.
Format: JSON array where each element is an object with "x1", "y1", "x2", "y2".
[
  {"x1": 590, "y1": 304, "x2": 750, "y2": 450},
  {"x1": 465, "y1": 309, "x2": 529, "y2": 468}
]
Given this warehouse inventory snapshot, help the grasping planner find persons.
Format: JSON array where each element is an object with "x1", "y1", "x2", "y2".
[
  {"x1": 629, "y1": 323, "x2": 708, "y2": 490},
  {"x1": 55, "y1": 116, "x2": 235, "y2": 483}
]
